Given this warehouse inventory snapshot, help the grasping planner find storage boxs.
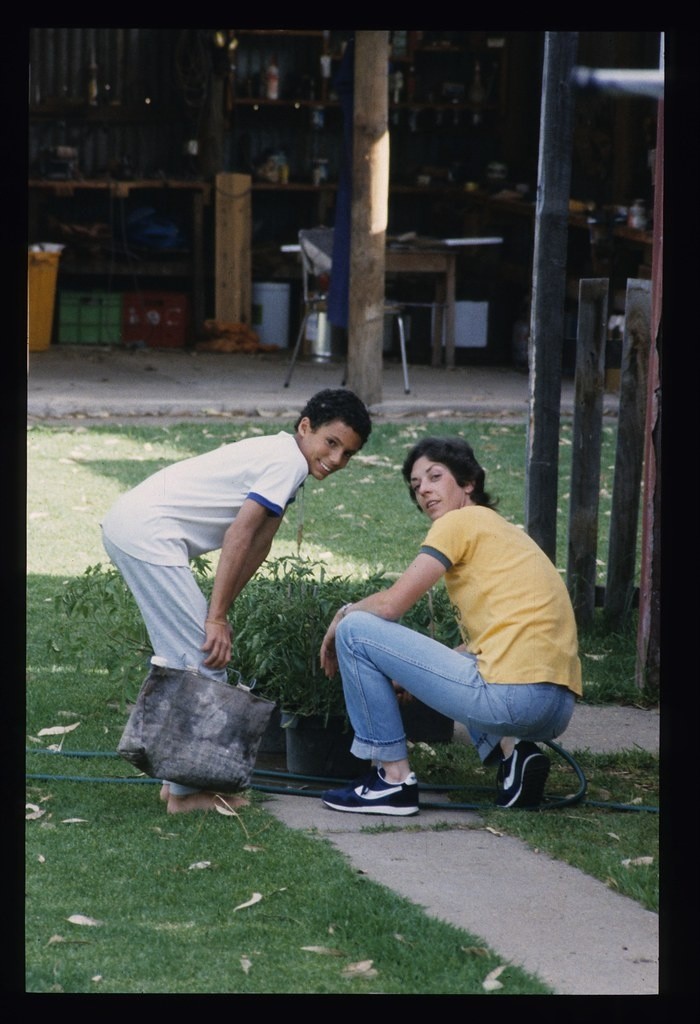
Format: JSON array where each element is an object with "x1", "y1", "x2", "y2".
[
  {"x1": 124, "y1": 291, "x2": 189, "y2": 347},
  {"x1": 57, "y1": 289, "x2": 123, "y2": 344}
]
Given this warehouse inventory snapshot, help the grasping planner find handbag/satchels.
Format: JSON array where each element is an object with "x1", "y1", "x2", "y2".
[{"x1": 116, "y1": 654, "x2": 277, "y2": 792}]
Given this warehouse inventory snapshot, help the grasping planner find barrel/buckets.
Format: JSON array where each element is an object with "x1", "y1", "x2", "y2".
[{"x1": 252, "y1": 281, "x2": 290, "y2": 348}]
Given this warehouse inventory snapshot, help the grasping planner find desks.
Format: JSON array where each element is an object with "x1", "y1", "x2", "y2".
[
  {"x1": 385, "y1": 244, "x2": 460, "y2": 373},
  {"x1": 464, "y1": 190, "x2": 651, "y2": 313}
]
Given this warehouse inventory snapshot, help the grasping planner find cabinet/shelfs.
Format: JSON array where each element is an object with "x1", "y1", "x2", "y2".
[
  {"x1": 27, "y1": 179, "x2": 212, "y2": 337},
  {"x1": 225, "y1": 29, "x2": 508, "y2": 190}
]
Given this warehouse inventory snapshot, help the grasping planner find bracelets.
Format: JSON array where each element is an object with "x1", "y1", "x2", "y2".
[
  {"x1": 341, "y1": 603, "x2": 352, "y2": 618},
  {"x1": 204, "y1": 619, "x2": 229, "y2": 626}
]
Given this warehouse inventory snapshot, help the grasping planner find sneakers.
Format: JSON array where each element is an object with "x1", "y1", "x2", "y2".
[
  {"x1": 492, "y1": 741, "x2": 552, "y2": 810},
  {"x1": 321, "y1": 764, "x2": 421, "y2": 816}
]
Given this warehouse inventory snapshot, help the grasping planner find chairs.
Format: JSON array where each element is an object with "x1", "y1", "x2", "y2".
[{"x1": 285, "y1": 226, "x2": 411, "y2": 394}]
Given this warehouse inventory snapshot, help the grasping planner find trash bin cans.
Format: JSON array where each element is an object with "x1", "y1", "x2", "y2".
[
  {"x1": 29, "y1": 241, "x2": 66, "y2": 352},
  {"x1": 253, "y1": 283, "x2": 291, "y2": 351}
]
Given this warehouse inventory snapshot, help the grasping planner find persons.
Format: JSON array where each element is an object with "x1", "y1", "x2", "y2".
[
  {"x1": 98, "y1": 388, "x2": 372, "y2": 814},
  {"x1": 320, "y1": 436, "x2": 583, "y2": 815}
]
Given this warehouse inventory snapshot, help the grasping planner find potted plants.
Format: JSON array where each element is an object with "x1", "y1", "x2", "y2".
[{"x1": 43, "y1": 555, "x2": 462, "y2": 778}]
